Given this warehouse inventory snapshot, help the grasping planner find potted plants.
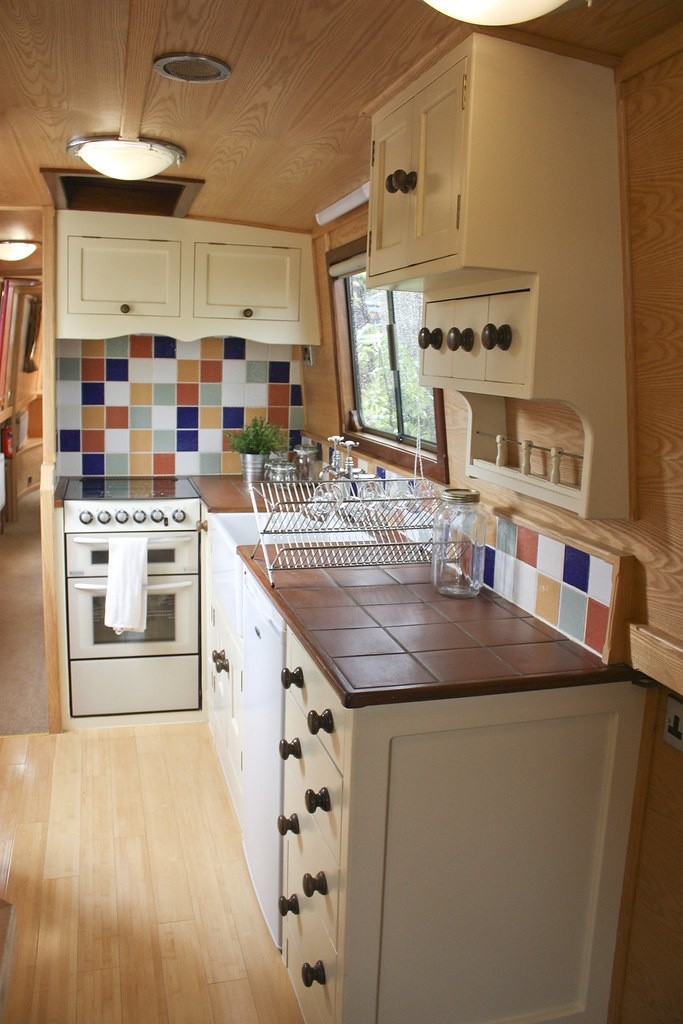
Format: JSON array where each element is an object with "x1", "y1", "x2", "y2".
[{"x1": 225, "y1": 417, "x2": 285, "y2": 482}]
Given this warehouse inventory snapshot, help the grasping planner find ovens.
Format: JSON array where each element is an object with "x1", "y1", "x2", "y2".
[{"x1": 63, "y1": 529, "x2": 202, "y2": 718}]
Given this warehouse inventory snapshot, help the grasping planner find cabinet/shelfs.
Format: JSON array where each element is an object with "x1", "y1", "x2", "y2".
[
  {"x1": 362, "y1": 22, "x2": 637, "y2": 521},
  {"x1": 198, "y1": 506, "x2": 644, "y2": 1024},
  {"x1": 55, "y1": 208, "x2": 321, "y2": 346}
]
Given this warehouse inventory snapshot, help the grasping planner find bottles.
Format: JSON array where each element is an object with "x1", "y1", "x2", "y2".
[
  {"x1": 431, "y1": 488, "x2": 487, "y2": 599},
  {"x1": 263, "y1": 444, "x2": 320, "y2": 490}
]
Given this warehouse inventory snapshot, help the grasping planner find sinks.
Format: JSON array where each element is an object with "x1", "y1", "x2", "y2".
[{"x1": 207, "y1": 506, "x2": 376, "y2": 547}]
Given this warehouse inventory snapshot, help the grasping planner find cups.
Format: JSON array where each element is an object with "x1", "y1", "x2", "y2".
[{"x1": 303, "y1": 477, "x2": 435, "y2": 521}]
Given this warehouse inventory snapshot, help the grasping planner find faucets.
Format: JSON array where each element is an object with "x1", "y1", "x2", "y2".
[{"x1": 319, "y1": 436, "x2": 359, "y2": 484}]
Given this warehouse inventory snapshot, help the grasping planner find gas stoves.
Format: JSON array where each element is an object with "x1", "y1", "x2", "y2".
[{"x1": 55, "y1": 475, "x2": 202, "y2": 534}]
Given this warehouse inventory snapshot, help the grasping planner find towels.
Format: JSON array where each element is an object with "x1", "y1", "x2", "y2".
[{"x1": 104, "y1": 537, "x2": 147, "y2": 632}]
